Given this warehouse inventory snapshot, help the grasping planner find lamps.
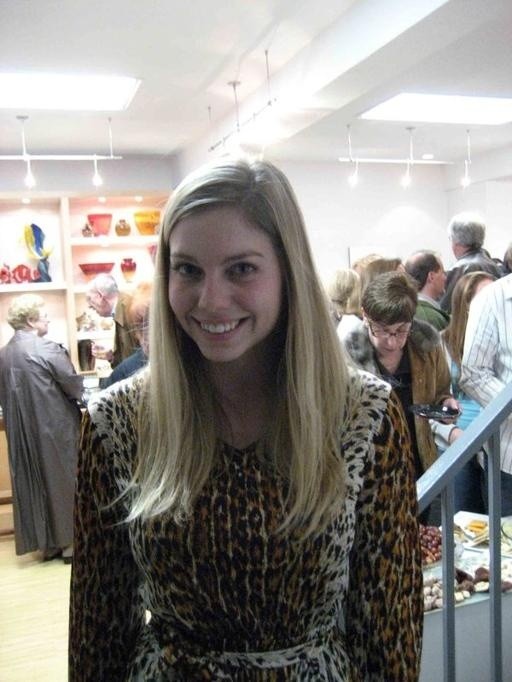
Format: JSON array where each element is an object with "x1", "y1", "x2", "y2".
[
  {"x1": 2, "y1": 120, "x2": 126, "y2": 188},
  {"x1": 332, "y1": 129, "x2": 475, "y2": 196}
]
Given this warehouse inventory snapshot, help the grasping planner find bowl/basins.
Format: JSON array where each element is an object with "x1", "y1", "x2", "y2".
[
  {"x1": 87, "y1": 213, "x2": 113, "y2": 238},
  {"x1": 132, "y1": 210, "x2": 160, "y2": 236},
  {"x1": 79, "y1": 262, "x2": 114, "y2": 278}
]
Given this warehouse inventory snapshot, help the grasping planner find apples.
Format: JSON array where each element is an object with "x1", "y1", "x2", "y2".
[{"x1": 419, "y1": 525, "x2": 442, "y2": 565}]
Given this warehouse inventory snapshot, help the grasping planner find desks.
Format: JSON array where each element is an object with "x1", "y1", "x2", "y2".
[{"x1": 409, "y1": 515, "x2": 512, "y2": 681}]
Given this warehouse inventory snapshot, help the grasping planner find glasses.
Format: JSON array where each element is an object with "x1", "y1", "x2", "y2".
[{"x1": 368, "y1": 317, "x2": 415, "y2": 338}]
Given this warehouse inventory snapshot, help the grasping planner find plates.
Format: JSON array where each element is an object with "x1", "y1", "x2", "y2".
[
  {"x1": 411, "y1": 403, "x2": 459, "y2": 419},
  {"x1": 415, "y1": 538, "x2": 463, "y2": 572},
  {"x1": 436, "y1": 508, "x2": 504, "y2": 552}
]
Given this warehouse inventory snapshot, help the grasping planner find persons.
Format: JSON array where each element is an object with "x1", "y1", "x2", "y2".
[
  {"x1": 0, "y1": 295, "x2": 83, "y2": 566},
  {"x1": 105, "y1": 292, "x2": 152, "y2": 388},
  {"x1": 326, "y1": 248, "x2": 512, "y2": 525},
  {"x1": 438, "y1": 211, "x2": 502, "y2": 315},
  {"x1": 84, "y1": 272, "x2": 141, "y2": 370},
  {"x1": 65, "y1": 152, "x2": 423, "y2": 682}
]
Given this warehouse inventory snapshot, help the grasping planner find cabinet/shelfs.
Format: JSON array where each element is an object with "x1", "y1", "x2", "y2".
[{"x1": 0, "y1": 189, "x2": 167, "y2": 537}]
